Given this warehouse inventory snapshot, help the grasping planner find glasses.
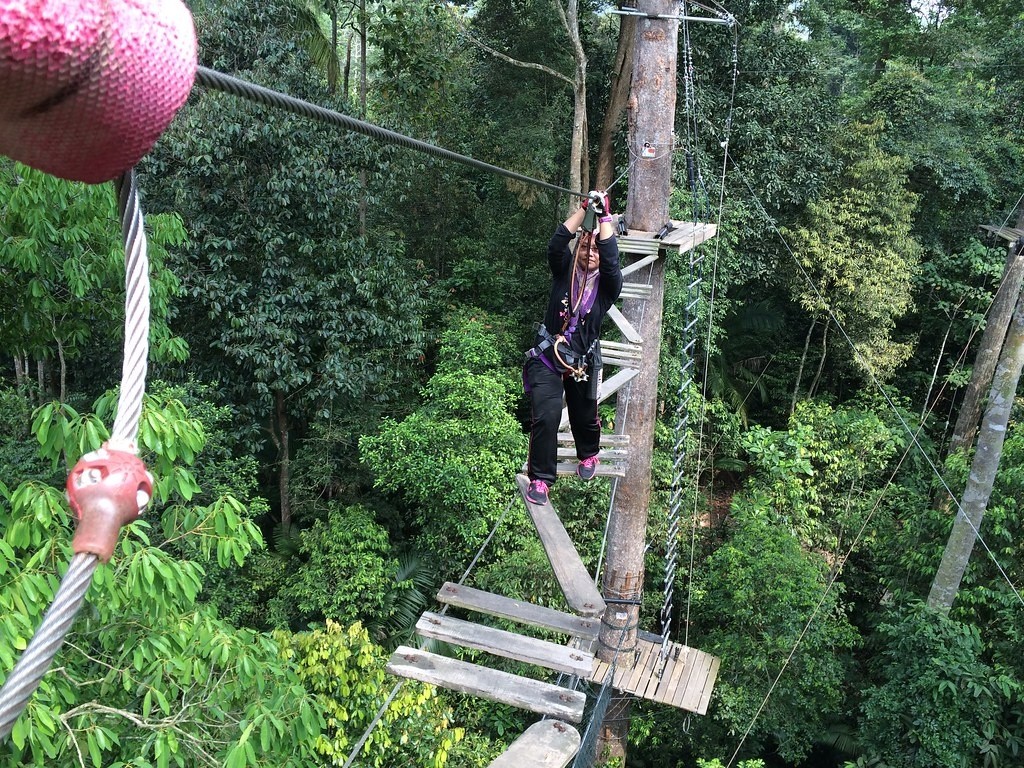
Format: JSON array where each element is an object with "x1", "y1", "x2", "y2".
[{"x1": 578, "y1": 243, "x2": 599, "y2": 253}]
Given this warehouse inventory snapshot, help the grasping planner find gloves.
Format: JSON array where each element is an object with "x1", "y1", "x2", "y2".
[{"x1": 581, "y1": 189, "x2": 609, "y2": 216}]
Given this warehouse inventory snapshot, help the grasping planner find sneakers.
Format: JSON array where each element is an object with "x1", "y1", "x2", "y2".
[
  {"x1": 577, "y1": 456, "x2": 600, "y2": 480},
  {"x1": 526, "y1": 478, "x2": 550, "y2": 506}
]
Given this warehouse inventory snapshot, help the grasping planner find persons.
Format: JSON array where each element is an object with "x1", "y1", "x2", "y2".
[{"x1": 526, "y1": 191, "x2": 623, "y2": 506}]
